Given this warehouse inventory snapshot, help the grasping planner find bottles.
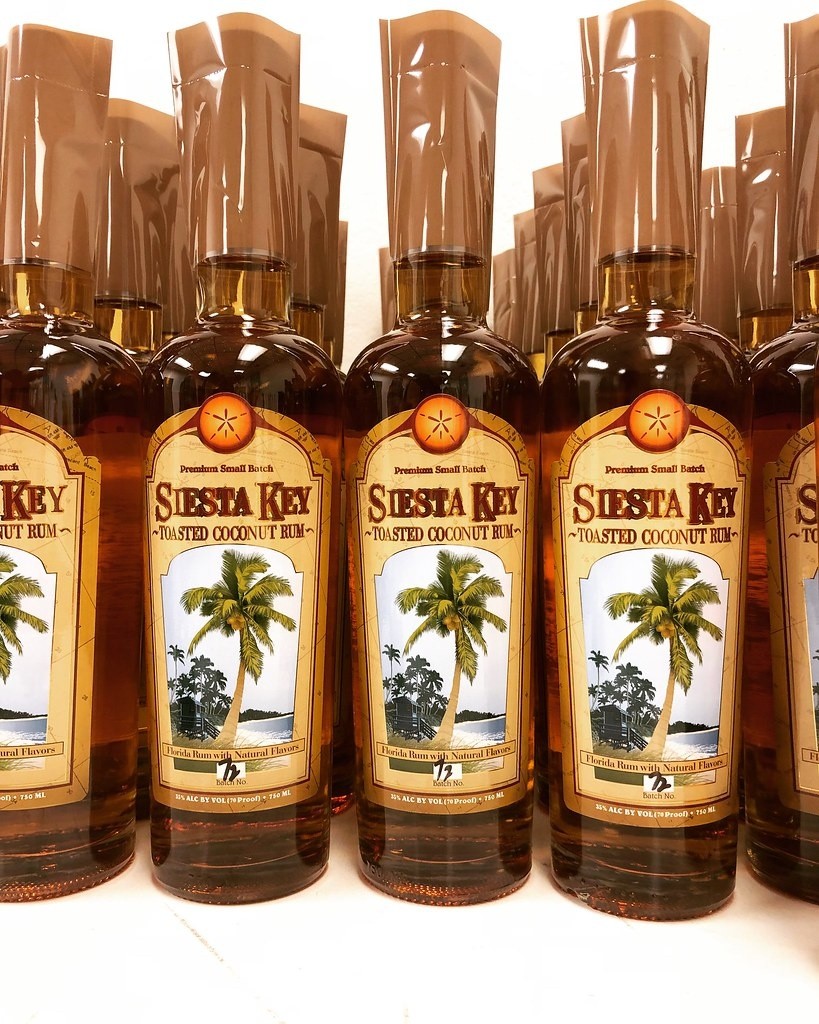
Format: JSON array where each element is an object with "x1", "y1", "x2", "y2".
[{"x1": 0, "y1": 0, "x2": 819, "y2": 922}]
